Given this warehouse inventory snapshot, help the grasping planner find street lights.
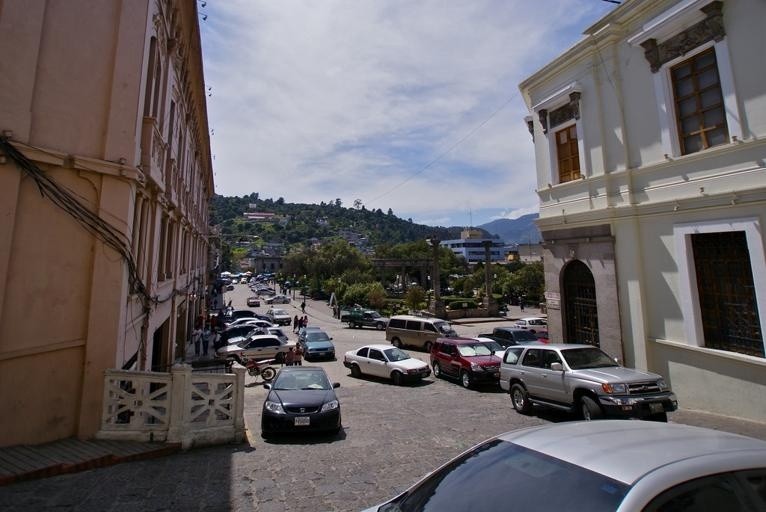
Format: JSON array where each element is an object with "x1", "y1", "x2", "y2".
[
  {"x1": 293, "y1": 273, "x2": 296, "y2": 299},
  {"x1": 280, "y1": 272, "x2": 282, "y2": 293},
  {"x1": 304, "y1": 274, "x2": 306, "y2": 304},
  {"x1": 337, "y1": 278, "x2": 341, "y2": 306}
]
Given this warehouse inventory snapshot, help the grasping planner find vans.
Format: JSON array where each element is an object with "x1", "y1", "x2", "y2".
[{"x1": 386, "y1": 314, "x2": 457, "y2": 352}]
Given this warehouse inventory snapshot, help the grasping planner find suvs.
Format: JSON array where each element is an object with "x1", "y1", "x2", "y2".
[{"x1": 500, "y1": 341, "x2": 678, "y2": 420}]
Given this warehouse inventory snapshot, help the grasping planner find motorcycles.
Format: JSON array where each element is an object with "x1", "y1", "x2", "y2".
[{"x1": 241, "y1": 354, "x2": 280, "y2": 383}]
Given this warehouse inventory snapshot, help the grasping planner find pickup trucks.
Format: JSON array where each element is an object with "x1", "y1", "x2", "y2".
[
  {"x1": 341, "y1": 310, "x2": 389, "y2": 330},
  {"x1": 217, "y1": 311, "x2": 270, "y2": 326}
]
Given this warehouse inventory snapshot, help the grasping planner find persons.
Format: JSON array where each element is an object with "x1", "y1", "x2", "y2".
[
  {"x1": 337, "y1": 305, "x2": 340, "y2": 319},
  {"x1": 333, "y1": 305, "x2": 337, "y2": 319},
  {"x1": 192, "y1": 300, "x2": 308, "y2": 366}
]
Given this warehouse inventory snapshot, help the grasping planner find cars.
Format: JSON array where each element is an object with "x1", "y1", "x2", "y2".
[
  {"x1": 266, "y1": 295, "x2": 291, "y2": 304},
  {"x1": 214, "y1": 317, "x2": 303, "y2": 367},
  {"x1": 266, "y1": 308, "x2": 291, "y2": 325},
  {"x1": 298, "y1": 327, "x2": 335, "y2": 361},
  {"x1": 344, "y1": 344, "x2": 431, "y2": 384},
  {"x1": 247, "y1": 297, "x2": 260, "y2": 307},
  {"x1": 431, "y1": 314, "x2": 548, "y2": 389},
  {"x1": 360, "y1": 420, "x2": 766, "y2": 512},
  {"x1": 262, "y1": 365, "x2": 341, "y2": 438},
  {"x1": 219, "y1": 270, "x2": 275, "y2": 295},
  {"x1": 513, "y1": 318, "x2": 548, "y2": 334}
]
